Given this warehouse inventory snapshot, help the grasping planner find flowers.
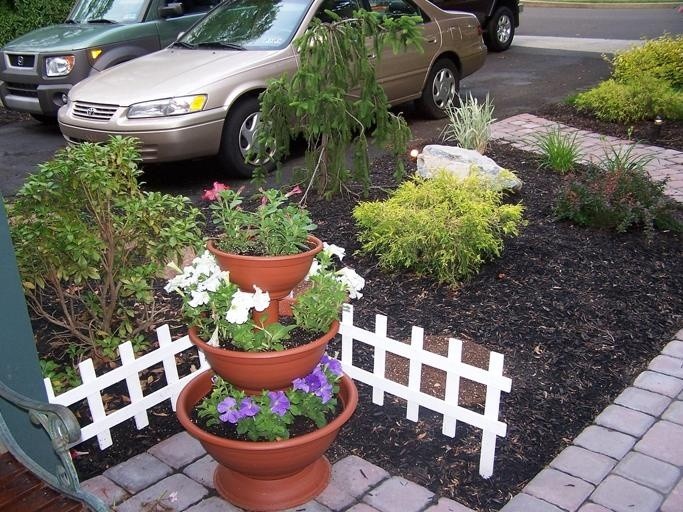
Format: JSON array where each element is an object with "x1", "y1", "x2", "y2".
[
  {"x1": 199, "y1": 184, "x2": 315, "y2": 253},
  {"x1": 204, "y1": 354, "x2": 342, "y2": 440},
  {"x1": 165, "y1": 240, "x2": 365, "y2": 350}
]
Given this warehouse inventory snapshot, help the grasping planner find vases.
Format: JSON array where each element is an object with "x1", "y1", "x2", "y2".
[
  {"x1": 207, "y1": 232, "x2": 327, "y2": 299},
  {"x1": 196, "y1": 302, "x2": 342, "y2": 394}
]
